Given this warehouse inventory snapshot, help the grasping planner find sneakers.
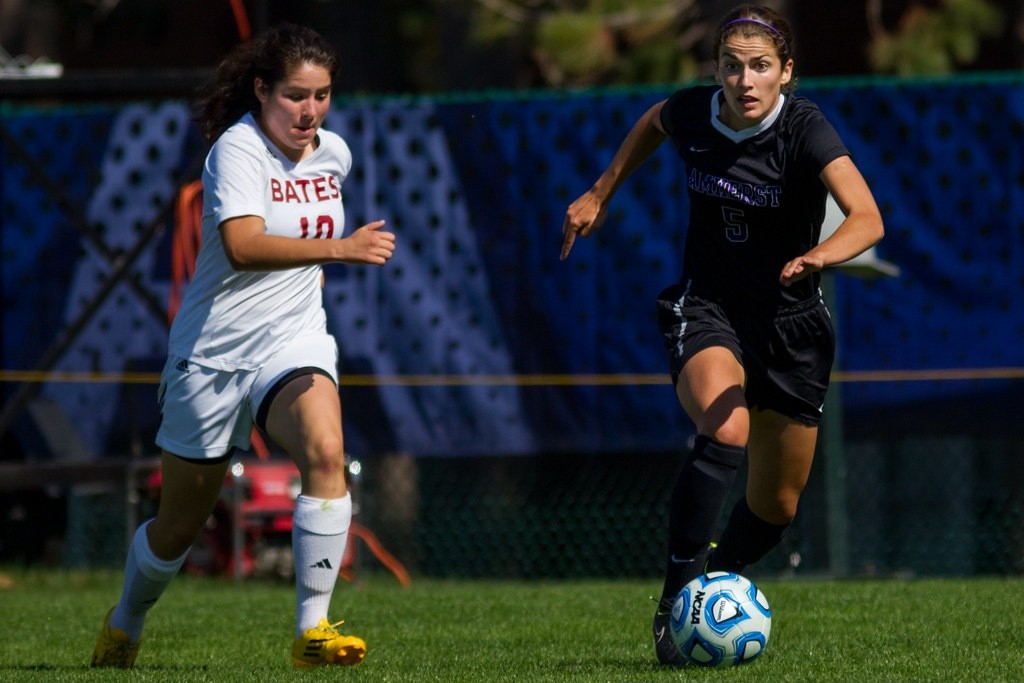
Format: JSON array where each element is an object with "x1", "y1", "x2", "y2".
[
  {"x1": 290, "y1": 620, "x2": 368, "y2": 667},
  {"x1": 652, "y1": 611, "x2": 686, "y2": 666},
  {"x1": 92, "y1": 604, "x2": 143, "y2": 668}
]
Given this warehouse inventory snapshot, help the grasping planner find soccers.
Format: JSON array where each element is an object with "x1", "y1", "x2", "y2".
[{"x1": 669, "y1": 570, "x2": 772, "y2": 668}]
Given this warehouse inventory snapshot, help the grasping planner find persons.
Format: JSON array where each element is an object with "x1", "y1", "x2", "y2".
[
  {"x1": 558, "y1": 2, "x2": 885, "y2": 669},
  {"x1": 92, "y1": 39, "x2": 395, "y2": 668}
]
